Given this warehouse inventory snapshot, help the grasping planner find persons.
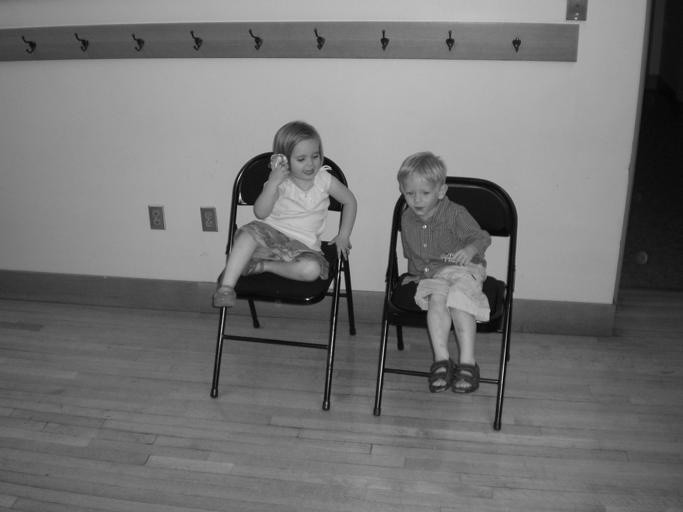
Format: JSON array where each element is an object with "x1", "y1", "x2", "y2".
[
  {"x1": 214, "y1": 121, "x2": 358, "y2": 307},
  {"x1": 397, "y1": 151, "x2": 491, "y2": 394}
]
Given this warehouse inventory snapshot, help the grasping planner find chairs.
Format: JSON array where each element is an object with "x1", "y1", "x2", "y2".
[
  {"x1": 210, "y1": 152, "x2": 355, "y2": 411},
  {"x1": 373, "y1": 176, "x2": 518, "y2": 430}
]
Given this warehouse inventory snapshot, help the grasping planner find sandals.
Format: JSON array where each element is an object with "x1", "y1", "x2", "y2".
[
  {"x1": 213, "y1": 256, "x2": 265, "y2": 307},
  {"x1": 428, "y1": 358, "x2": 480, "y2": 394}
]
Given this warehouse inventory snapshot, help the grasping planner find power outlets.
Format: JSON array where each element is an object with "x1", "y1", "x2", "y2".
[
  {"x1": 200, "y1": 207, "x2": 218, "y2": 231},
  {"x1": 149, "y1": 205, "x2": 164, "y2": 230}
]
